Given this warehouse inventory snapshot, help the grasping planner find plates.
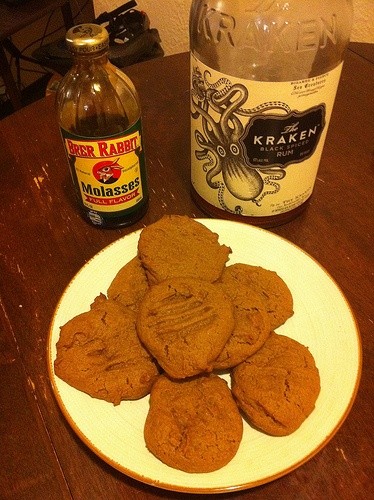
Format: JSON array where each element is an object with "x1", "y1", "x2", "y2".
[{"x1": 46, "y1": 218, "x2": 363, "y2": 494}]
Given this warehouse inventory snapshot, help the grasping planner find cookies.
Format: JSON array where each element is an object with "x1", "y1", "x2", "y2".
[{"x1": 53, "y1": 215, "x2": 321, "y2": 472}]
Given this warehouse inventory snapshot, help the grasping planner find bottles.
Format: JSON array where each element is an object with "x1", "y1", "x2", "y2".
[
  {"x1": 54, "y1": 24, "x2": 149, "y2": 229},
  {"x1": 190, "y1": 0, "x2": 354, "y2": 229}
]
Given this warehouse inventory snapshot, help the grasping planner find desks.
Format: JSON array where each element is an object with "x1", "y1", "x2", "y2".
[{"x1": 0, "y1": 42, "x2": 373, "y2": 500}]
